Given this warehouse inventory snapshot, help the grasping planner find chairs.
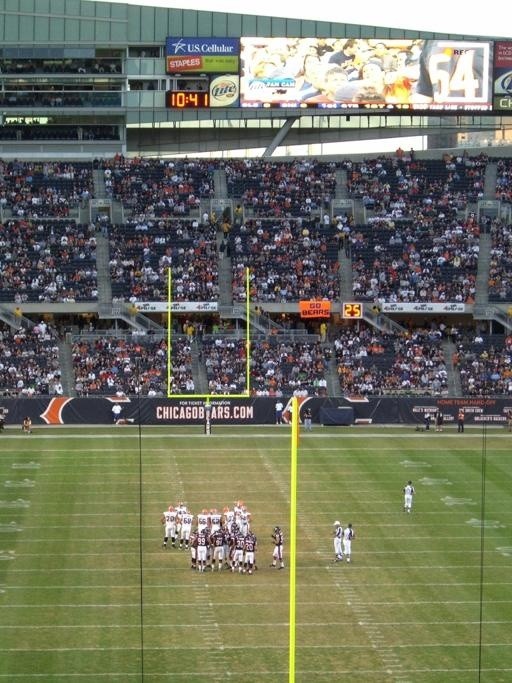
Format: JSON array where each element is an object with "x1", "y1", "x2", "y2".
[{"x1": 1, "y1": 150, "x2": 512, "y2": 396}]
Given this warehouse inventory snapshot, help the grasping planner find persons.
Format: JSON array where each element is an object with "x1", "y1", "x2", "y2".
[
  {"x1": 343, "y1": 524, "x2": 355, "y2": 563},
  {"x1": 268, "y1": 526, "x2": 287, "y2": 570},
  {"x1": 0, "y1": 147, "x2": 512, "y2": 432},
  {"x1": 161, "y1": 500, "x2": 258, "y2": 574},
  {"x1": 401, "y1": 481, "x2": 415, "y2": 511},
  {"x1": 330, "y1": 520, "x2": 343, "y2": 564},
  {"x1": 241, "y1": 37, "x2": 490, "y2": 108}
]
